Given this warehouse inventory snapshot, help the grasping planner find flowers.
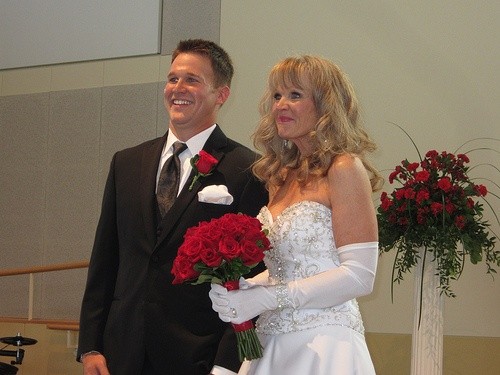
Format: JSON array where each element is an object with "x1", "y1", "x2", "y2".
[
  {"x1": 368, "y1": 122, "x2": 500, "y2": 334},
  {"x1": 188, "y1": 150, "x2": 220, "y2": 190},
  {"x1": 171, "y1": 213, "x2": 273, "y2": 363}
]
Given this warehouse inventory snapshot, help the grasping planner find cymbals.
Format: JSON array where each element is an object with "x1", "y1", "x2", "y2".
[{"x1": 0, "y1": 336, "x2": 38, "y2": 346}]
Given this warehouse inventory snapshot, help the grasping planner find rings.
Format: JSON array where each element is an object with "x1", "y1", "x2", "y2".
[{"x1": 232, "y1": 308, "x2": 237, "y2": 315}]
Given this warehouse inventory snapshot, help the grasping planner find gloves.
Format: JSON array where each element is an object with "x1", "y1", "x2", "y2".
[
  {"x1": 211, "y1": 242, "x2": 379, "y2": 324},
  {"x1": 208, "y1": 269, "x2": 269, "y2": 322}
]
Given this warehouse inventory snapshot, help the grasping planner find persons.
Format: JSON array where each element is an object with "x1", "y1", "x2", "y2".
[
  {"x1": 209, "y1": 57, "x2": 384, "y2": 375},
  {"x1": 76, "y1": 39, "x2": 270, "y2": 375}
]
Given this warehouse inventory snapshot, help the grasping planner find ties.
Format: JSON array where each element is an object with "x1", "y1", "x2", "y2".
[{"x1": 156, "y1": 142, "x2": 188, "y2": 218}]
